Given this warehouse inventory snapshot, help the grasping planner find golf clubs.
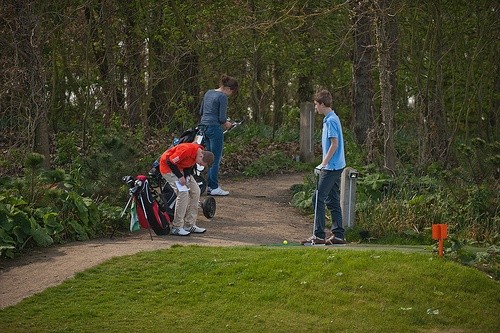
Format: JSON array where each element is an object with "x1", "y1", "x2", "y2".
[{"x1": 310, "y1": 174, "x2": 319, "y2": 246}]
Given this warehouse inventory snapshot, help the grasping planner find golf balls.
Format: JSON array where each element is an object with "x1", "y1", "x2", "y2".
[{"x1": 283, "y1": 240, "x2": 288, "y2": 245}]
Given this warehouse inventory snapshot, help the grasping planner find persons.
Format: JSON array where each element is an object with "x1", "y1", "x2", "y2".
[
  {"x1": 300, "y1": 89, "x2": 348, "y2": 246},
  {"x1": 160, "y1": 143, "x2": 214, "y2": 236},
  {"x1": 199, "y1": 74, "x2": 238, "y2": 196}
]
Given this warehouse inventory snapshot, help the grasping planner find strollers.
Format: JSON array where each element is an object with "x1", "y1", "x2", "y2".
[{"x1": 124, "y1": 125, "x2": 216, "y2": 233}]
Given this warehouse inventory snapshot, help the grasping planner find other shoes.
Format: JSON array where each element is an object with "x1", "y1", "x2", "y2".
[
  {"x1": 207, "y1": 188, "x2": 230, "y2": 196},
  {"x1": 301, "y1": 236, "x2": 326, "y2": 247},
  {"x1": 325, "y1": 235, "x2": 346, "y2": 247},
  {"x1": 170, "y1": 227, "x2": 191, "y2": 236},
  {"x1": 183, "y1": 225, "x2": 206, "y2": 233}
]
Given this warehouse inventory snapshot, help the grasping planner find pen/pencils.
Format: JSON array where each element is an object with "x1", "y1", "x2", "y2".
[{"x1": 185, "y1": 184, "x2": 189, "y2": 188}]
